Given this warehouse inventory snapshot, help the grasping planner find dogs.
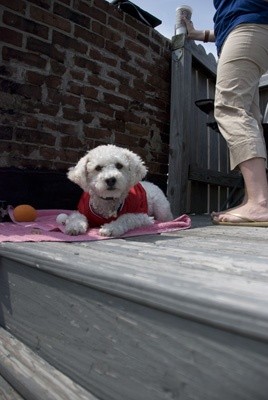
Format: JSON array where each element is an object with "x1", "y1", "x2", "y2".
[{"x1": 55, "y1": 144, "x2": 174, "y2": 238}]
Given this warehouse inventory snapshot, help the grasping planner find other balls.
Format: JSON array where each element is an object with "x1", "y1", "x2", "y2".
[
  {"x1": 56, "y1": 213, "x2": 67, "y2": 225},
  {"x1": 14, "y1": 205, "x2": 37, "y2": 222}
]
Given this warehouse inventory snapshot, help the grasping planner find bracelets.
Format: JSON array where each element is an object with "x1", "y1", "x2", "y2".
[{"x1": 203, "y1": 29, "x2": 211, "y2": 43}]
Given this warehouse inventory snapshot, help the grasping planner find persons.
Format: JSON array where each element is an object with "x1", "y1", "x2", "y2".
[{"x1": 181, "y1": 0, "x2": 268, "y2": 227}]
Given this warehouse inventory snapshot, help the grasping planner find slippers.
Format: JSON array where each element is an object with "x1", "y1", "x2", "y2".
[{"x1": 211, "y1": 208, "x2": 268, "y2": 228}]
